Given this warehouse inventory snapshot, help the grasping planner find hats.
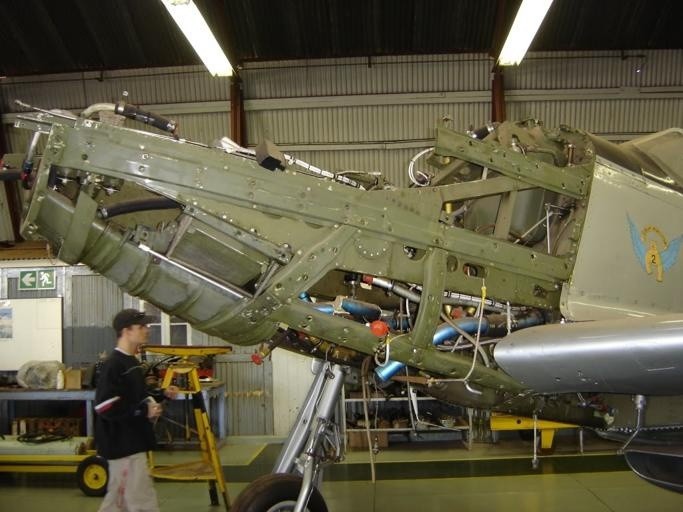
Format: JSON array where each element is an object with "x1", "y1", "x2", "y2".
[{"x1": 111, "y1": 308, "x2": 157, "y2": 329}]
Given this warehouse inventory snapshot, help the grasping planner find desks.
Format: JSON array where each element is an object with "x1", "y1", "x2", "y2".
[{"x1": 149, "y1": 379, "x2": 227, "y2": 451}]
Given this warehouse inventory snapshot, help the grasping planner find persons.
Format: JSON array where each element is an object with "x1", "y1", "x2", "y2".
[{"x1": 95, "y1": 309, "x2": 160, "y2": 511}]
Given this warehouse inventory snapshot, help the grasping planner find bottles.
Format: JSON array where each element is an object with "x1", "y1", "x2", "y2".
[
  {"x1": 11, "y1": 416, "x2": 44, "y2": 437},
  {"x1": 55, "y1": 369, "x2": 65, "y2": 390}
]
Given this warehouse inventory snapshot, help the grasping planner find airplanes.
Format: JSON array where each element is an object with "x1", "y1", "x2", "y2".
[{"x1": 1, "y1": 99, "x2": 683, "y2": 511}]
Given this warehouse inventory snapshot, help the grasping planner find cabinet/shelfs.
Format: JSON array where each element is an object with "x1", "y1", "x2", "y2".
[{"x1": 331, "y1": 381, "x2": 475, "y2": 456}]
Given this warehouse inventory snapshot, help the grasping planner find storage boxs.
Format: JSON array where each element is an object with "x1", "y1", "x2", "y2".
[{"x1": 347, "y1": 430, "x2": 389, "y2": 449}]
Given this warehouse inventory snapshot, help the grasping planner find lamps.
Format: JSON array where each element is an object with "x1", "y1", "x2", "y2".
[
  {"x1": 490, "y1": 1, "x2": 555, "y2": 69},
  {"x1": 158, "y1": 1, "x2": 239, "y2": 81}
]
